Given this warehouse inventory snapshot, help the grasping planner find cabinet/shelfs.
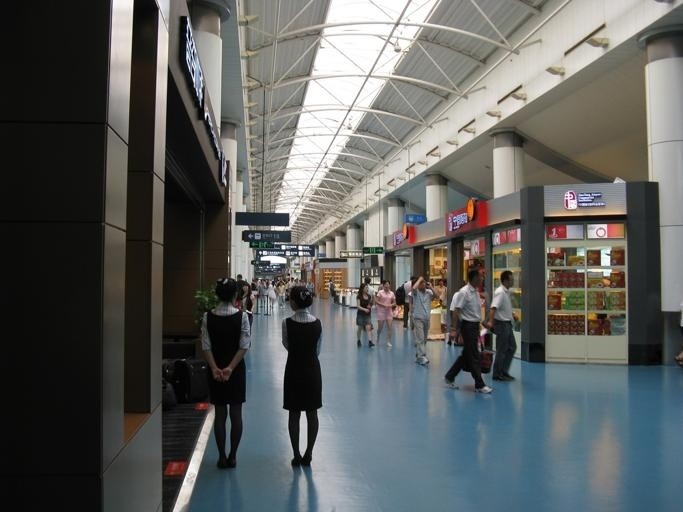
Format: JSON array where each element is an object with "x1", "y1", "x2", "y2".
[
  {"x1": 429, "y1": 248, "x2": 448, "y2": 308},
  {"x1": 321, "y1": 268, "x2": 343, "y2": 295},
  {"x1": 491, "y1": 245, "x2": 628, "y2": 364}
]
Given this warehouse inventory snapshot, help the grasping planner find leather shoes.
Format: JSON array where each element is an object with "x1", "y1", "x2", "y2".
[{"x1": 492, "y1": 373, "x2": 514, "y2": 381}]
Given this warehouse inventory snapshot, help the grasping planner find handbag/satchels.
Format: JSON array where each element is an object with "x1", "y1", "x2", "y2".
[
  {"x1": 395, "y1": 285, "x2": 405, "y2": 306},
  {"x1": 462, "y1": 349, "x2": 494, "y2": 374}
]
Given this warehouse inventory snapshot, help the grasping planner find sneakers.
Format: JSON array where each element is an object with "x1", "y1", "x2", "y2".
[
  {"x1": 474, "y1": 385, "x2": 493, "y2": 394},
  {"x1": 358, "y1": 339, "x2": 393, "y2": 348},
  {"x1": 415, "y1": 358, "x2": 429, "y2": 365},
  {"x1": 217, "y1": 456, "x2": 237, "y2": 470},
  {"x1": 444, "y1": 377, "x2": 459, "y2": 389},
  {"x1": 292, "y1": 453, "x2": 313, "y2": 467}
]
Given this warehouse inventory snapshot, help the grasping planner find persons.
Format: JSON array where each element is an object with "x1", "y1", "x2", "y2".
[
  {"x1": 252, "y1": 273, "x2": 315, "y2": 315},
  {"x1": 200, "y1": 277, "x2": 251, "y2": 469},
  {"x1": 442, "y1": 264, "x2": 493, "y2": 394},
  {"x1": 355, "y1": 274, "x2": 447, "y2": 366},
  {"x1": 239, "y1": 282, "x2": 255, "y2": 348},
  {"x1": 282, "y1": 285, "x2": 322, "y2": 467},
  {"x1": 486, "y1": 270, "x2": 520, "y2": 381},
  {"x1": 236, "y1": 274, "x2": 244, "y2": 290}
]
